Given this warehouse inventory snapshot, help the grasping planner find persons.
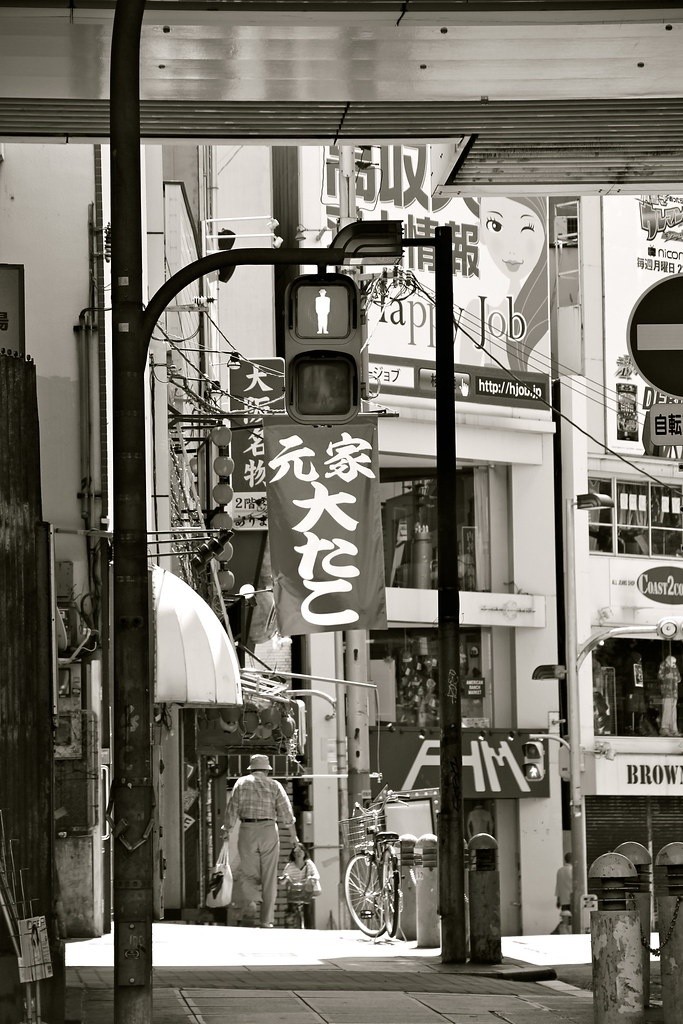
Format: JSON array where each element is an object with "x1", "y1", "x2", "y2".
[
  {"x1": 658, "y1": 655, "x2": 682, "y2": 737},
  {"x1": 221, "y1": 754, "x2": 300, "y2": 927},
  {"x1": 279, "y1": 843, "x2": 321, "y2": 923},
  {"x1": 624, "y1": 654, "x2": 649, "y2": 715},
  {"x1": 555, "y1": 852, "x2": 573, "y2": 923},
  {"x1": 465, "y1": 804, "x2": 494, "y2": 839}
]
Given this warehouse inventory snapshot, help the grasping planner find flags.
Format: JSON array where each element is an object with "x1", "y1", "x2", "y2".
[{"x1": 262, "y1": 413, "x2": 388, "y2": 636}]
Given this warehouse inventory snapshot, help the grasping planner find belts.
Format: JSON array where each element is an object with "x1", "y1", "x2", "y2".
[{"x1": 241, "y1": 819, "x2": 272, "y2": 823}]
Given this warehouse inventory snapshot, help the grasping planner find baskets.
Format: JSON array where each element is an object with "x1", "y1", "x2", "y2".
[{"x1": 338, "y1": 814, "x2": 376, "y2": 847}]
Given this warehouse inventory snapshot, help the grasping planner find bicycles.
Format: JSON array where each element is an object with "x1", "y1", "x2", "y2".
[{"x1": 336, "y1": 787, "x2": 413, "y2": 944}]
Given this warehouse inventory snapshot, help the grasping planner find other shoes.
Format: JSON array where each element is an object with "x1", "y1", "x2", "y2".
[
  {"x1": 261, "y1": 922, "x2": 274, "y2": 929},
  {"x1": 247, "y1": 901, "x2": 256, "y2": 918}
]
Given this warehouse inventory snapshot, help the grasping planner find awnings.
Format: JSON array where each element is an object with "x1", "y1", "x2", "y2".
[{"x1": 151, "y1": 566, "x2": 243, "y2": 710}]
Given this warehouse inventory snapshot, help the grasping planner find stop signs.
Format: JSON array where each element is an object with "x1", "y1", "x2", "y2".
[{"x1": 627, "y1": 273, "x2": 683, "y2": 402}]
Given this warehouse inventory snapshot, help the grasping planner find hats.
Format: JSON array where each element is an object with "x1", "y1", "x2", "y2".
[{"x1": 247, "y1": 754, "x2": 272, "y2": 770}]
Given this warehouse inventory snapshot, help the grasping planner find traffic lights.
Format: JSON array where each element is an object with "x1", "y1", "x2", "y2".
[
  {"x1": 521, "y1": 741, "x2": 545, "y2": 781},
  {"x1": 285, "y1": 270, "x2": 368, "y2": 424}
]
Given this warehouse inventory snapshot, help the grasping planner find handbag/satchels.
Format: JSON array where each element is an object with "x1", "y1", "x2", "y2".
[
  {"x1": 312, "y1": 878, "x2": 321, "y2": 896},
  {"x1": 205, "y1": 839, "x2": 233, "y2": 908},
  {"x1": 288, "y1": 882, "x2": 311, "y2": 903}
]
